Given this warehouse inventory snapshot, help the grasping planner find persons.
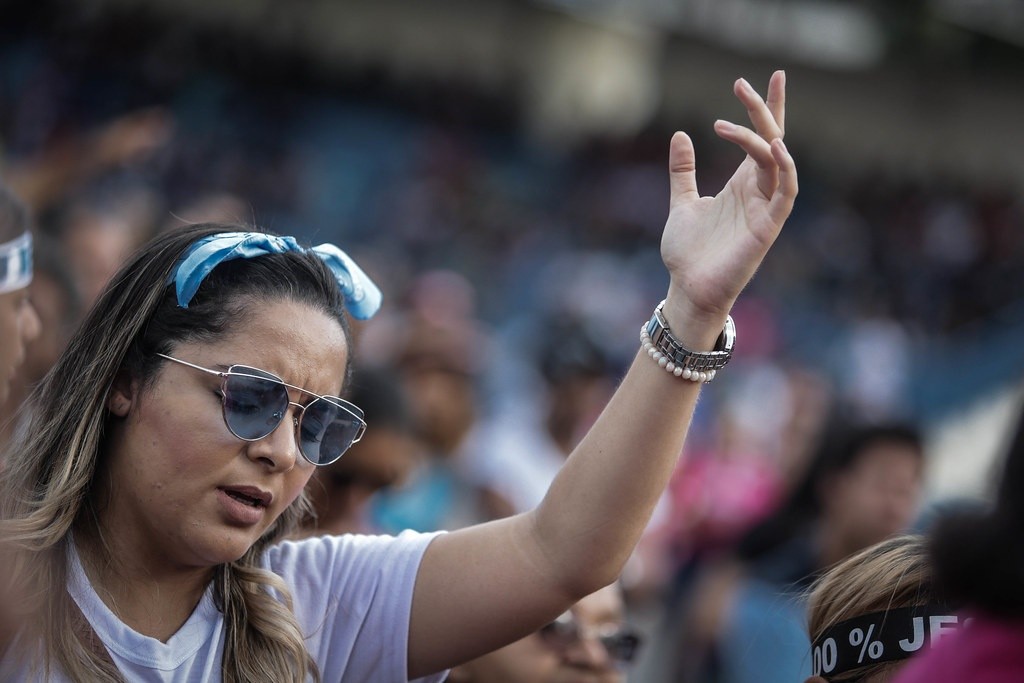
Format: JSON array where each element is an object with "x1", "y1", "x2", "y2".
[
  {"x1": 0, "y1": 110, "x2": 1024, "y2": 683},
  {"x1": 0, "y1": 68, "x2": 800, "y2": 681}
]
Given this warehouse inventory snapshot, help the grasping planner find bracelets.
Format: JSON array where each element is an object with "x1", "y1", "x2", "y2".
[
  {"x1": 646, "y1": 297, "x2": 736, "y2": 371},
  {"x1": 639, "y1": 321, "x2": 716, "y2": 382}
]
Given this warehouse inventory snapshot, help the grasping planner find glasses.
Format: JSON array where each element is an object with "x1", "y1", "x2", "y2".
[
  {"x1": 536, "y1": 600, "x2": 645, "y2": 663},
  {"x1": 146, "y1": 352, "x2": 374, "y2": 468}
]
